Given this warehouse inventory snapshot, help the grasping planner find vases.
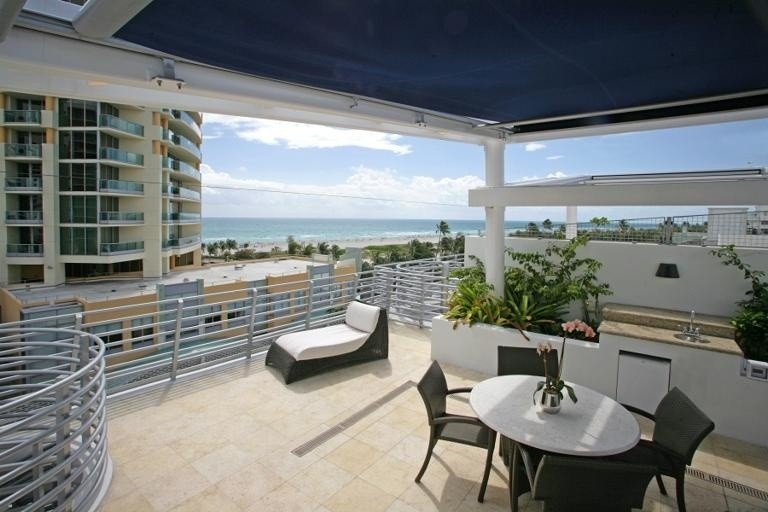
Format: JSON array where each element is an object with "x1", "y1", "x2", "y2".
[{"x1": 540, "y1": 392, "x2": 561, "y2": 413}]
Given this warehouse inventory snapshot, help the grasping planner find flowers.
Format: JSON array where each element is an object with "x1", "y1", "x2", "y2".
[{"x1": 533, "y1": 338, "x2": 578, "y2": 406}]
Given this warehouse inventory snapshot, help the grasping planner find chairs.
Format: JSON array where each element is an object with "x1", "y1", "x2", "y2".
[
  {"x1": 416, "y1": 361, "x2": 497, "y2": 502},
  {"x1": 497, "y1": 345, "x2": 560, "y2": 456},
  {"x1": 507, "y1": 445, "x2": 657, "y2": 511},
  {"x1": 627, "y1": 385, "x2": 714, "y2": 512},
  {"x1": 266, "y1": 300, "x2": 389, "y2": 385}
]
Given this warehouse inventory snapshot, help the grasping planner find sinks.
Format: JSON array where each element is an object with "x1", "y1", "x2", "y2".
[{"x1": 673, "y1": 334, "x2": 710, "y2": 343}]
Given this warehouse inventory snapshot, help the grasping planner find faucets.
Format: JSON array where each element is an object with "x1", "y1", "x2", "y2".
[{"x1": 683, "y1": 310, "x2": 700, "y2": 337}]
